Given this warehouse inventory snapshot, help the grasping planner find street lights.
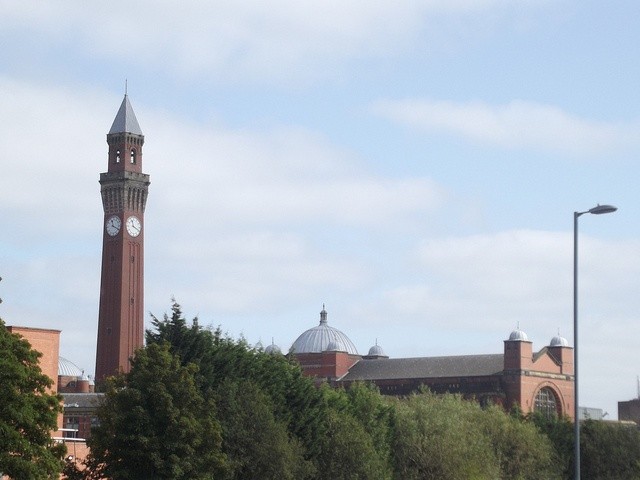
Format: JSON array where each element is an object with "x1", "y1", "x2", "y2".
[{"x1": 574, "y1": 204, "x2": 616, "y2": 480}]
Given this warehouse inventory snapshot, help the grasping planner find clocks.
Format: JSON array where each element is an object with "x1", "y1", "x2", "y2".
[
  {"x1": 104, "y1": 214, "x2": 122, "y2": 237},
  {"x1": 126, "y1": 215, "x2": 143, "y2": 238}
]
